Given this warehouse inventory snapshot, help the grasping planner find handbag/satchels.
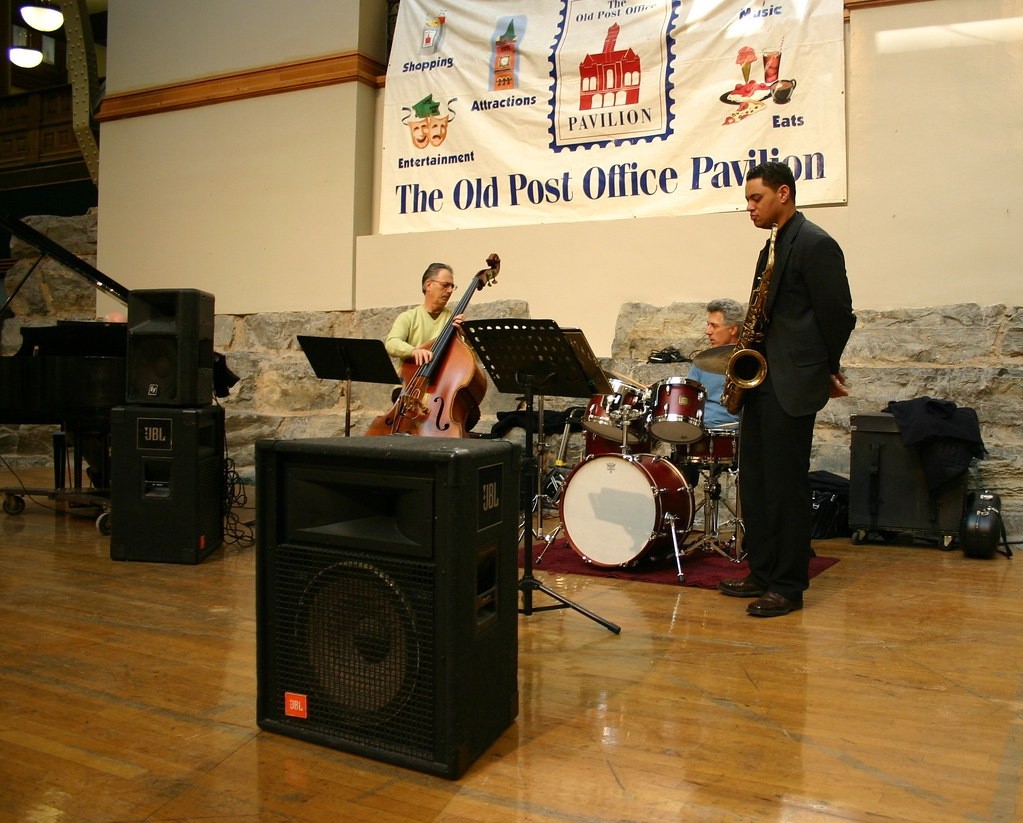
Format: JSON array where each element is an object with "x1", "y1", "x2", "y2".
[{"x1": 808, "y1": 470, "x2": 850, "y2": 538}]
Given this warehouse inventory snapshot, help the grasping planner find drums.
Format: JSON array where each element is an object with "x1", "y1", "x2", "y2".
[
  {"x1": 677, "y1": 427, "x2": 740, "y2": 468},
  {"x1": 646, "y1": 375, "x2": 710, "y2": 446},
  {"x1": 580, "y1": 376, "x2": 653, "y2": 445},
  {"x1": 560, "y1": 452, "x2": 698, "y2": 571}
]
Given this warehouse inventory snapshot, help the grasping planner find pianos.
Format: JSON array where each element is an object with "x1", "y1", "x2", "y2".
[{"x1": 0, "y1": 319, "x2": 244, "y2": 490}]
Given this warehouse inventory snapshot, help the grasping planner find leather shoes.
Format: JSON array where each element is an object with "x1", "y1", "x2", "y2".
[
  {"x1": 719, "y1": 575, "x2": 765, "y2": 595},
  {"x1": 748, "y1": 590, "x2": 803, "y2": 616}
]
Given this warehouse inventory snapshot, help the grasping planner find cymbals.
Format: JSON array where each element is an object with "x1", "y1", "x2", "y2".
[{"x1": 692, "y1": 343, "x2": 737, "y2": 375}]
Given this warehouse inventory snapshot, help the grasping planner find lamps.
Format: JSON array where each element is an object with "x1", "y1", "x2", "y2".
[{"x1": 9, "y1": 5, "x2": 64, "y2": 69}]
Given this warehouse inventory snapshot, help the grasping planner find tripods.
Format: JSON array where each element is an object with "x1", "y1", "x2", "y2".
[
  {"x1": 684, "y1": 467, "x2": 746, "y2": 563},
  {"x1": 518, "y1": 328, "x2": 615, "y2": 541},
  {"x1": 462, "y1": 319, "x2": 621, "y2": 634}
]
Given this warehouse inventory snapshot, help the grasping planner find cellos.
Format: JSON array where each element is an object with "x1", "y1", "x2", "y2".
[{"x1": 363, "y1": 250, "x2": 501, "y2": 438}]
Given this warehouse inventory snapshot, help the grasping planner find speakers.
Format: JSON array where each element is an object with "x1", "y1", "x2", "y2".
[
  {"x1": 110, "y1": 404, "x2": 225, "y2": 565},
  {"x1": 254, "y1": 434, "x2": 523, "y2": 782},
  {"x1": 125, "y1": 288, "x2": 215, "y2": 407}
]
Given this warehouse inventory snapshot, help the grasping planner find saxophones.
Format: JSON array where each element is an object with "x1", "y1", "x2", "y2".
[{"x1": 719, "y1": 220, "x2": 780, "y2": 417}]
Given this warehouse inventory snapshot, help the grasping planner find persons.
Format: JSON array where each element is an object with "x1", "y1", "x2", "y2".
[
  {"x1": 385, "y1": 263, "x2": 481, "y2": 431},
  {"x1": 686, "y1": 162, "x2": 857, "y2": 617}
]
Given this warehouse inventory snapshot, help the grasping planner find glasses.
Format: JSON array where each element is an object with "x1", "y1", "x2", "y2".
[{"x1": 431, "y1": 280, "x2": 457, "y2": 290}]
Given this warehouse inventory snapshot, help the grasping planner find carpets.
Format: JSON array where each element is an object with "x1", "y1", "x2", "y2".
[{"x1": 518, "y1": 536, "x2": 840, "y2": 591}]
